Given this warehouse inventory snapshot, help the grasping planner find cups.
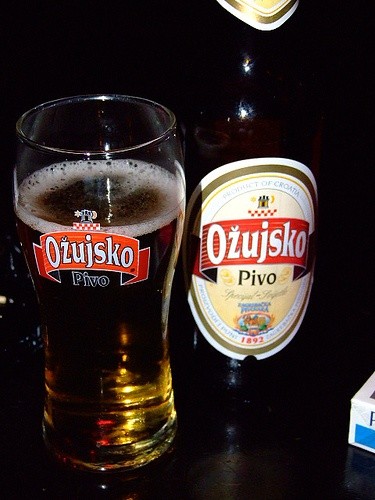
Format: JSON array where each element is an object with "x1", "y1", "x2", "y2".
[{"x1": 15, "y1": 91, "x2": 187, "y2": 475}]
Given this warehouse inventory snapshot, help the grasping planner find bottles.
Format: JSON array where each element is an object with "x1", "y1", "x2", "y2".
[{"x1": 180, "y1": 0, "x2": 329, "y2": 437}]
